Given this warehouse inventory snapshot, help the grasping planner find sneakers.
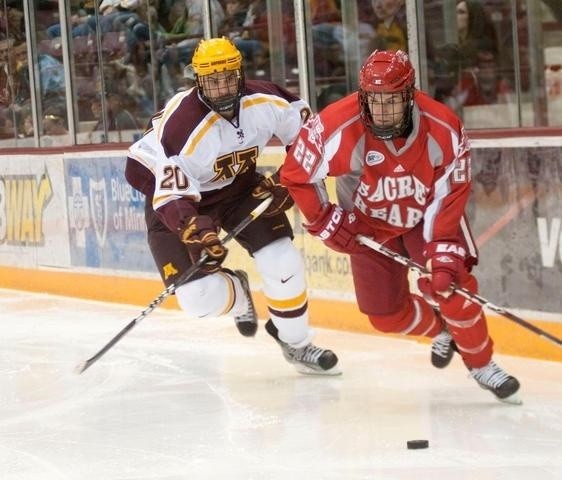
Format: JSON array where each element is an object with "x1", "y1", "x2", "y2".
[
  {"x1": 431, "y1": 333, "x2": 453, "y2": 368},
  {"x1": 289, "y1": 343, "x2": 337, "y2": 370},
  {"x1": 470, "y1": 359, "x2": 520, "y2": 399},
  {"x1": 233, "y1": 270, "x2": 257, "y2": 336}
]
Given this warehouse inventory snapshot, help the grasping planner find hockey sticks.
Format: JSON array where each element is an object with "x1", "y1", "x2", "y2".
[{"x1": 72, "y1": 194, "x2": 273, "y2": 375}]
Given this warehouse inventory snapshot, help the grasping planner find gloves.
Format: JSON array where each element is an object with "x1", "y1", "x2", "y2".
[
  {"x1": 417, "y1": 241, "x2": 466, "y2": 305},
  {"x1": 180, "y1": 215, "x2": 228, "y2": 272},
  {"x1": 248, "y1": 166, "x2": 295, "y2": 217},
  {"x1": 304, "y1": 203, "x2": 375, "y2": 254}
]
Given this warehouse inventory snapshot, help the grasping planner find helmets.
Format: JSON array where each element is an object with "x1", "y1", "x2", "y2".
[
  {"x1": 359, "y1": 49, "x2": 415, "y2": 139},
  {"x1": 192, "y1": 36, "x2": 244, "y2": 112}
]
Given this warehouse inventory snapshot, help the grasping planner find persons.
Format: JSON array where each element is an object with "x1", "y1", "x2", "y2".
[
  {"x1": 0, "y1": 0, "x2": 562, "y2": 140},
  {"x1": 280, "y1": 49, "x2": 520, "y2": 398},
  {"x1": 124, "y1": 36, "x2": 338, "y2": 371}
]
